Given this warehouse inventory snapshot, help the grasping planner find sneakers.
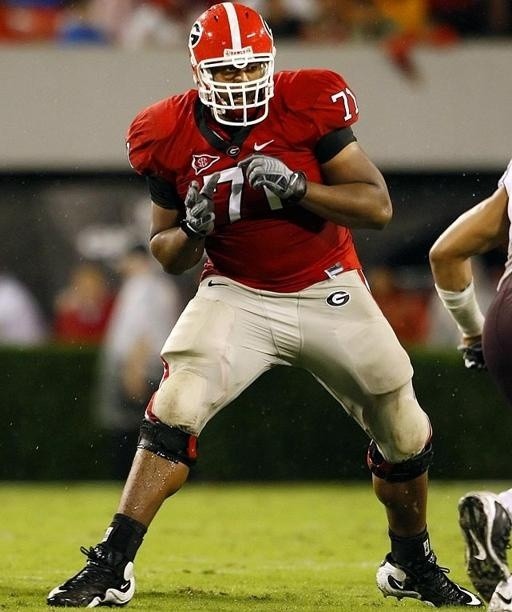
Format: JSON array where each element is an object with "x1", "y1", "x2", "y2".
[
  {"x1": 458, "y1": 490, "x2": 512, "y2": 611},
  {"x1": 47, "y1": 539, "x2": 136, "y2": 609},
  {"x1": 375, "y1": 551, "x2": 485, "y2": 610}
]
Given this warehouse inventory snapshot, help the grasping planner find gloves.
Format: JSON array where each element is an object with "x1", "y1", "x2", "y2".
[
  {"x1": 236, "y1": 154, "x2": 309, "y2": 207},
  {"x1": 459, "y1": 335, "x2": 488, "y2": 370},
  {"x1": 179, "y1": 172, "x2": 221, "y2": 241}
]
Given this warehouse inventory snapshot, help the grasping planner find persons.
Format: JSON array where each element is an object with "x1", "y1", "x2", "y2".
[
  {"x1": 424, "y1": 154, "x2": 511, "y2": 610},
  {"x1": 86, "y1": 232, "x2": 188, "y2": 478},
  {"x1": 2, "y1": 1, "x2": 465, "y2": 48},
  {"x1": 45, "y1": 1, "x2": 488, "y2": 608},
  {"x1": 2, "y1": 242, "x2": 502, "y2": 355}
]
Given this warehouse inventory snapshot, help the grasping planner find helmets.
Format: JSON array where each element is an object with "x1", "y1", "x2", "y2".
[{"x1": 187, "y1": 2, "x2": 277, "y2": 128}]
